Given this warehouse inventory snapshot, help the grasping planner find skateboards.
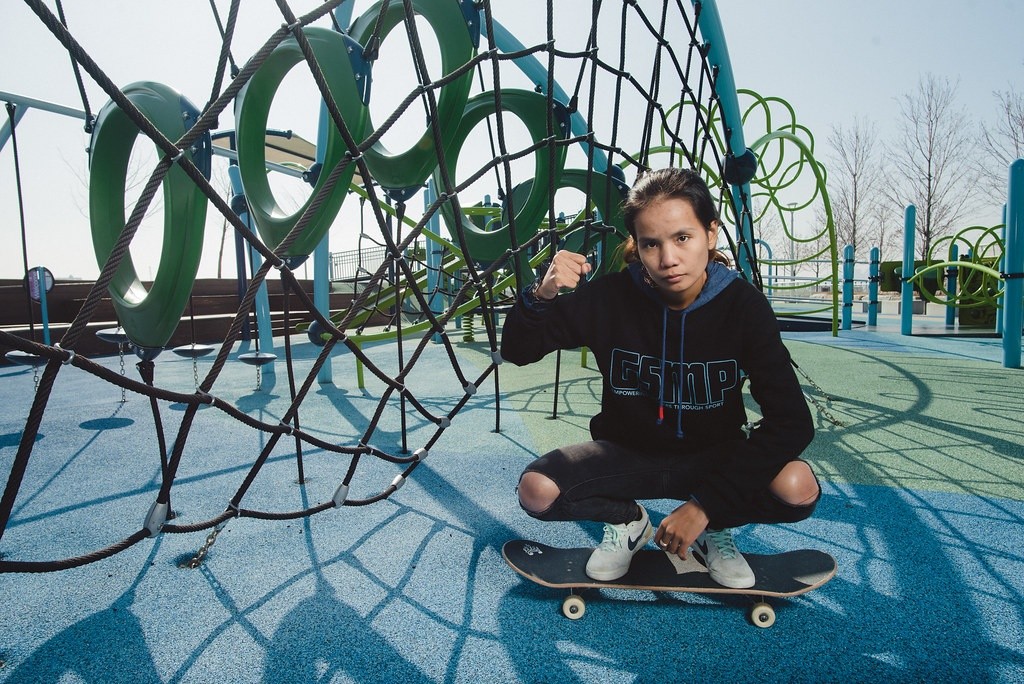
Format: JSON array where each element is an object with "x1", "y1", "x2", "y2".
[{"x1": 501, "y1": 538, "x2": 840, "y2": 629}]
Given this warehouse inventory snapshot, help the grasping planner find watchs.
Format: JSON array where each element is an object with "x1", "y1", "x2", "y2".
[{"x1": 527, "y1": 278, "x2": 558, "y2": 304}]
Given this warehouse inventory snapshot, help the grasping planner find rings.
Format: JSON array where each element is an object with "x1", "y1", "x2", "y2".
[{"x1": 660, "y1": 539, "x2": 670, "y2": 547}]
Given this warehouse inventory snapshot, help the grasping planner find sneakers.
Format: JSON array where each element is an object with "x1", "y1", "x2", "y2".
[
  {"x1": 585, "y1": 504, "x2": 653, "y2": 582},
  {"x1": 691, "y1": 527, "x2": 756, "y2": 589}
]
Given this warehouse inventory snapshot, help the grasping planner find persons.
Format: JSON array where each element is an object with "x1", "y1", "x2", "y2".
[{"x1": 501, "y1": 169, "x2": 821, "y2": 589}]
